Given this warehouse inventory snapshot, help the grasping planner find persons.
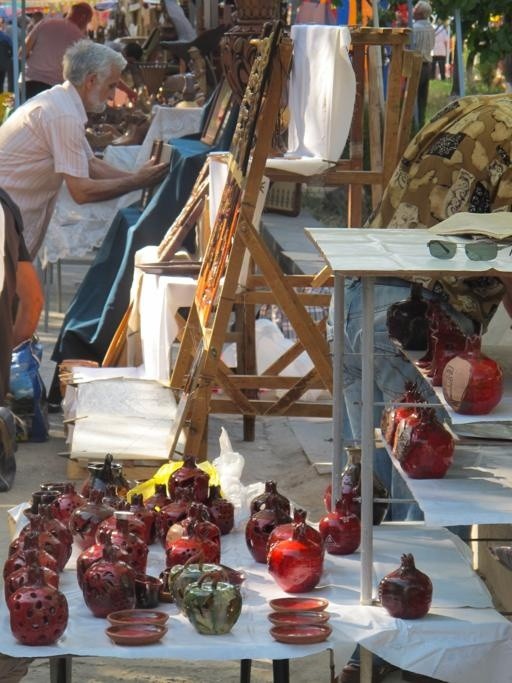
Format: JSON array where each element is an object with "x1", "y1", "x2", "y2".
[
  {"x1": 367, "y1": 0, "x2": 450, "y2": 139},
  {"x1": 1, "y1": 1, "x2": 169, "y2": 411}
]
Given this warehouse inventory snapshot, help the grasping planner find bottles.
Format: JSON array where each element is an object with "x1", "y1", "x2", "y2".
[
  {"x1": 440, "y1": 334, "x2": 505, "y2": 416},
  {"x1": 3, "y1": 452, "x2": 332, "y2": 646},
  {"x1": 318, "y1": 496, "x2": 361, "y2": 555},
  {"x1": 380, "y1": 378, "x2": 455, "y2": 480},
  {"x1": 379, "y1": 552, "x2": 434, "y2": 618},
  {"x1": 324, "y1": 444, "x2": 390, "y2": 525}
]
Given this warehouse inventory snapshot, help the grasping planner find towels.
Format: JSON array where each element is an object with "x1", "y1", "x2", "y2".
[{"x1": 248, "y1": 24, "x2": 357, "y2": 176}]
